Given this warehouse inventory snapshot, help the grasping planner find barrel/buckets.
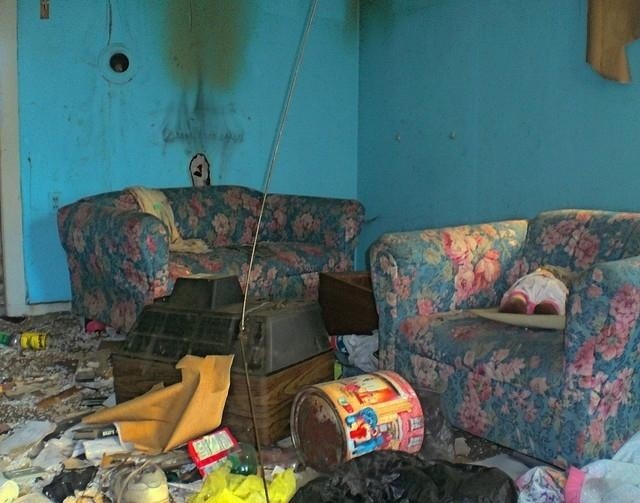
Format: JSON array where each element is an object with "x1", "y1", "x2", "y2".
[{"x1": 288, "y1": 371, "x2": 424, "y2": 474}]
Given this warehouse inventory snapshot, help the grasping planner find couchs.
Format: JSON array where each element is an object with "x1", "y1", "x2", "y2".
[
  {"x1": 55, "y1": 185, "x2": 363, "y2": 336},
  {"x1": 372, "y1": 209, "x2": 640, "y2": 471}
]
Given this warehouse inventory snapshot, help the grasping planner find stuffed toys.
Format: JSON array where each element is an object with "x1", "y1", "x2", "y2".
[{"x1": 500, "y1": 265, "x2": 576, "y2": 315}]
